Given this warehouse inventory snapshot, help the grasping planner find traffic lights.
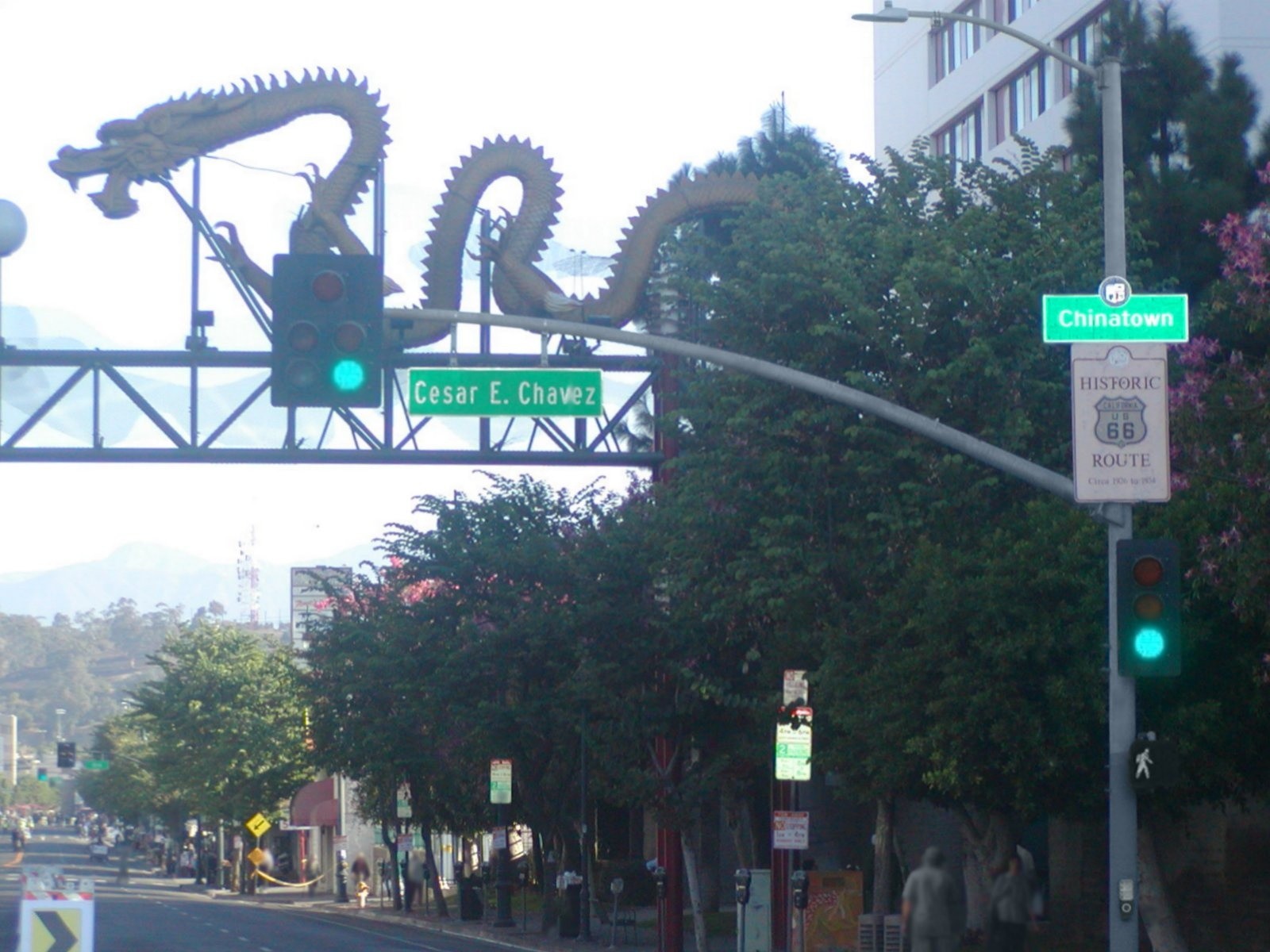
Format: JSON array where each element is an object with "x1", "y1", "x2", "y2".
[
  {"x1": 270, "y1": 251, "x2": 386, "y2": 411},
  {"x1": 37, "y1": 768, "x2": 48, "y2": 782},
  {"x1": 57, "y1": 741, "x2": 76, "y2": 769},
  {"x1": 1116, "y1": 538, "x2": 1186, "y2": 682}
]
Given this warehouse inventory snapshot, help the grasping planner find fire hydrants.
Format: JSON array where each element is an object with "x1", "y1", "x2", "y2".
[{"x1": 356, "y1": 881, "x2": 372, "y2": 909}]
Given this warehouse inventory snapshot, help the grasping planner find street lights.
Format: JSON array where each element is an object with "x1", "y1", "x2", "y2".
[{"x1": 850, "y1": 2, "x2": 1139, "y2": 952}]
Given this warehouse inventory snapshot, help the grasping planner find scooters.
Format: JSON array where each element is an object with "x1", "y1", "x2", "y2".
[{"x1": 13, "y1": 832, "x2": 24, "y2": 850}]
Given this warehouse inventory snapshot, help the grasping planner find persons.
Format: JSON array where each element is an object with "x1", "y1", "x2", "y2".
[
  {"x1": 900, "y1": 847, "x2": 961, "y2": 952},
  {"x1": 351, "y1": 853, "x2": 370, "y2": 899},
  {"x1": 407, "y1": 852, "x2": 424, "y2": 907},
  {"x1": 983, "y1": 831, "x2": 1042, "y2": 952},
  {"x1": 259, "y1": 843, "x2": 274, "y2": 888}
]
